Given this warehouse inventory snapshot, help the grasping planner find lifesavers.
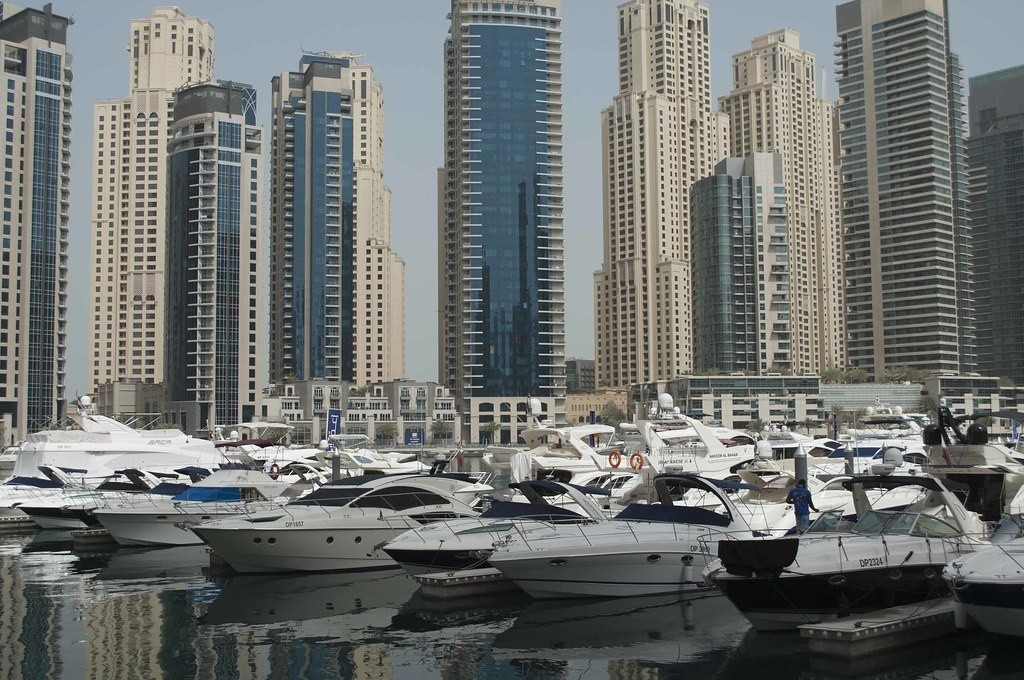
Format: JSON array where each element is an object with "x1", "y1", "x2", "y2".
[
  {"x1": 608, "y1": 450, "x2": 622, "y2": 468},
  {"x1": 630, "y1": 452, "x2": 644, "y2": 470},
  {"x1": 270, "y1": 463, "x2": 280, "y2": 480}
]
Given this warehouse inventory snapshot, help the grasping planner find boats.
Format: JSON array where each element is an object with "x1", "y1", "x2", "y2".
[{"x1": 0, "y1": 418, "x2": 1024, "y2": 641}]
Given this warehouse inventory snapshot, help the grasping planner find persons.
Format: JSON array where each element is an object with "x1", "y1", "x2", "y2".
[{"x1": 786, "y1": 479, "x2": 819, "y2": 536}]
